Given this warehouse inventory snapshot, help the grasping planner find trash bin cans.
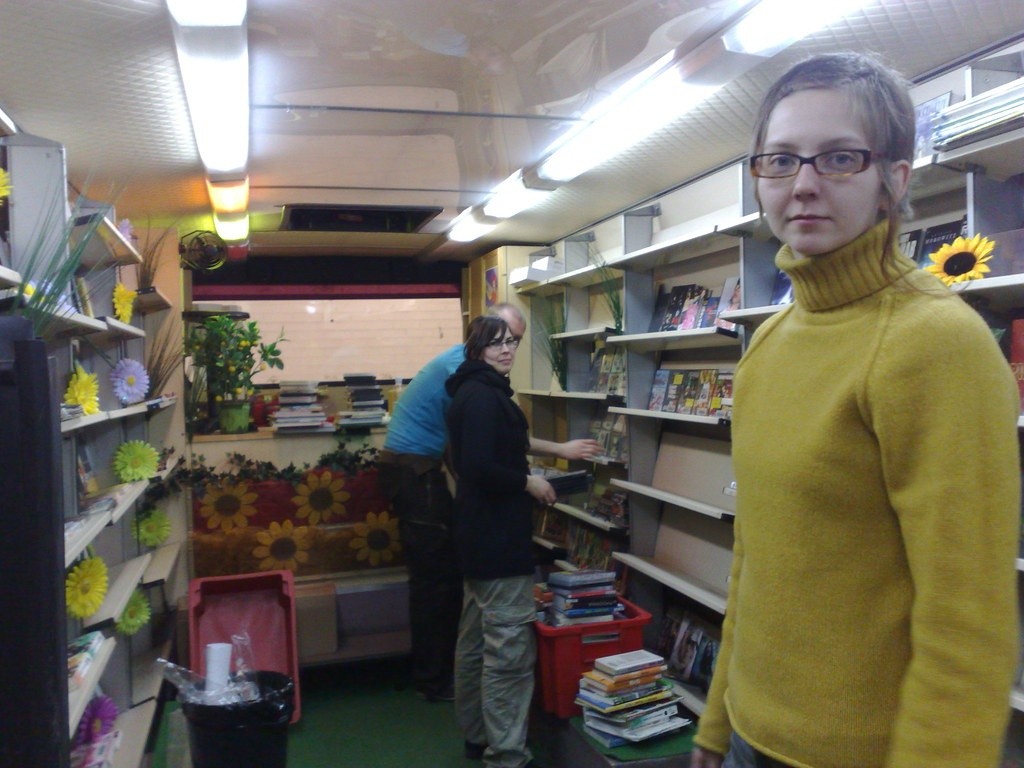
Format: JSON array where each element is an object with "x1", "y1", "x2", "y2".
[{"x1": 176, "y1": 670, "x2": 296, "y2": 768}]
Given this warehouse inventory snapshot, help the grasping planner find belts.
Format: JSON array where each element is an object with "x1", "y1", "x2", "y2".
[{"x1": 379, "y1": 450, "x2": 434, "y2": 465}]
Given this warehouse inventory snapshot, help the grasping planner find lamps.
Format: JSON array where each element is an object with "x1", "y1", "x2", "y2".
[
  {"x1": 168, "y1": 0, "x2": 251, "y2": 245},
  {"x1": 447, "y1": 0, "x2": 876, "y2": 244}
]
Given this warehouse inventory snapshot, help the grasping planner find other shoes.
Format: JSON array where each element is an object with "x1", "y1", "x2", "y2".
[
  {"x1": 465, "y1": 740, "x2": 491, "y2": 759},
  {"x1": 525, "y1": 758, "x2": 546, "y2": 768},
  {"x1": 416, "y1": 687, "x2": 426, "y2": 697},
  {"x1": 426, "y1": 687, "x2": 456, "y2": 700}
]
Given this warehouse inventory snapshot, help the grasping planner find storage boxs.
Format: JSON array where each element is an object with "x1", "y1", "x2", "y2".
[
  {"x1": 532, "y1": 592, "x2": 654, "y2": 719},
  {"x1": 509, "y1": 266, "x2": 560, "y2": 288},
  {"x1": 533, "y1": 256, "x2": 564, "y2": 272}
]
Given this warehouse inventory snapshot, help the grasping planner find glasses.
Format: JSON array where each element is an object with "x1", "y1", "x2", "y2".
[
  {"x1": 750, "y1": 147, "x2": 888, "y2": 179},
  {"x1": 485, "y1": 338, "x2": 516, "y2": 349}
]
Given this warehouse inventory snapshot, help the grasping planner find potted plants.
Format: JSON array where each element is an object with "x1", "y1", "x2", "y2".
[{"x1": 180, "y1": 312, "x2": 291, "y2": 434}]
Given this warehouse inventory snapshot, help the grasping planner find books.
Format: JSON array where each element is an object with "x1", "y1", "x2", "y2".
[
  {"x1": 530, "y1": 467, "x2": 595, "y2": 497},
  {"x1": 769, "y1": 268, "x2": 795, "y2": 305},
  {"x1": 896, "y1": 216, "x2": 967, "y2": 270},
  {"x1": 658, "y1": 277, "x2": 741, "y2": 332},
  {"x1": 139, "y1": 398, "x2": 162, "y2": 406},
  {"x1": 534, "y1": 568, "x2": 625, "y2": 627},
  {"x1": 76, "y1": 447, "x2": 128, "y2": 515},
  {"x1": 68, "y1": 631, "x2": 104, "y2": 691},
  {"x1": 337, "y1": 375, "x2": 390, "y2": 425},
  {"x1": 271, "y1": 379, "x2": 336, "y2": 433},
  {"x1": 914, "y1": 90, "x2": 965, "y2": 159},
  {"x1": 573, "y1": 649, "x2": 691, "y2": 748},
  {"x1": 158, "y1": 454, "x2": 175, "y2": 471},
  {"x1": 71, "y1": 276, "x2": 93, "y2": 316},
  {"x1": 653, "y1": 609, "x2": 720, "y2": 696},
  {"x1": 587, "y1": 341, "x2": 628, "y2": 464},
  {"x1": 929, "y1": 76, "x2": 1024, "y2": 152},
  {"x1": 648, "y1": 368, "x2": 734, "y2": 421}
]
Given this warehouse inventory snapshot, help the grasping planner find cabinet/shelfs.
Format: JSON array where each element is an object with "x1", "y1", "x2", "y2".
[
  {"x1": 0, "y1": 213, "x2": 180, "y2": 768},
  {"x1": 517, "y1": 51, "x2": 1024, "y2": 718},
  {"x1": 186, "y1": 378, "x2": 412, "y2": 691}
]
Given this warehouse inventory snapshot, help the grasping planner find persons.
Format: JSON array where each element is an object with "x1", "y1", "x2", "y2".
[
  {"x1": 377, "y1": 302, "x2": 607, "y2": 703},
  {"x1": 685, "y1": 52, "x2": 1024, "y2": 768},
  {"x1": 443, "y1": 315, "x2": 557, "y2": 767}
]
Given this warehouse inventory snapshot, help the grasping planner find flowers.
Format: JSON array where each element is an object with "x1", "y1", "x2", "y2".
[
  {"x1": 923, "y1": 232, "x2": 996, "y2": 285},
  {"x1": 0, "y1": 159, "x2": 403, "y2": 738}
]
